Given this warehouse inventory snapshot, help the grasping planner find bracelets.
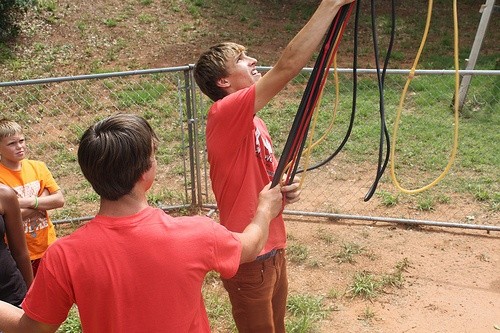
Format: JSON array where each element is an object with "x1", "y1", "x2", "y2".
[{"x1": 31, "y1": 196, "x2": 38, "y2": 210}]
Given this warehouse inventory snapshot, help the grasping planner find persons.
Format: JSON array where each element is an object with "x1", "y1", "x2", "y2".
[
  {"x1": 0, "y1": 182, "x2": 36, "y2": 310},
  {"x1": 193, "y1": 0, "x2": 359, "y2": 333},
  {"x1": 0, "y1": 118, "x2": 66, "y2": 279},
  {"x1": 0, "y1": 113, "x2": 282, "y2": 333}
]
